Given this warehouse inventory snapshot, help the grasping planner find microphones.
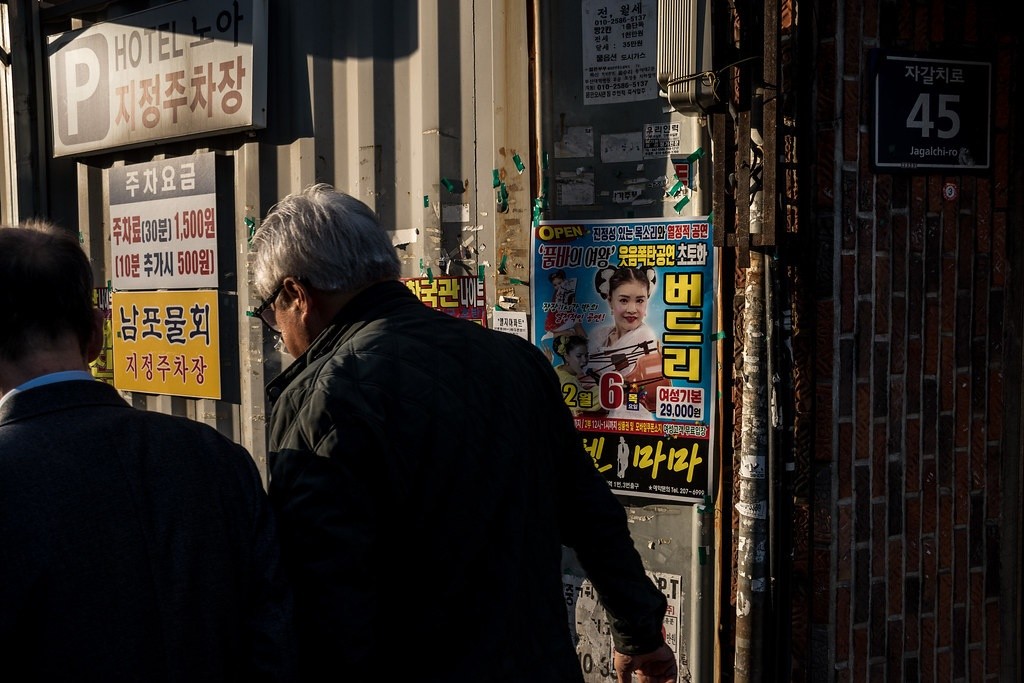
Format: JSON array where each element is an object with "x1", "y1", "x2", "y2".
[{"x1": 582, "y1": 367, "x2": 601, "y2": 386}]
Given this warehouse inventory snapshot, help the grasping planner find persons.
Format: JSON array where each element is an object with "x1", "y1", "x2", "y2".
[
  {"x1": 542, "y1": 264, "x2": 676, "y2": 423},
  {"x1": 250, "y1": 184, "x2": 680, "y2": 683},
  {"x1": 0, "y1": 220, "x2": 296, "y2": 683}
]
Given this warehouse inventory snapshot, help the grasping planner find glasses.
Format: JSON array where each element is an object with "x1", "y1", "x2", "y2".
[{"x1": 253, "y1": 274, "x2": 311, "y2": 336}]
